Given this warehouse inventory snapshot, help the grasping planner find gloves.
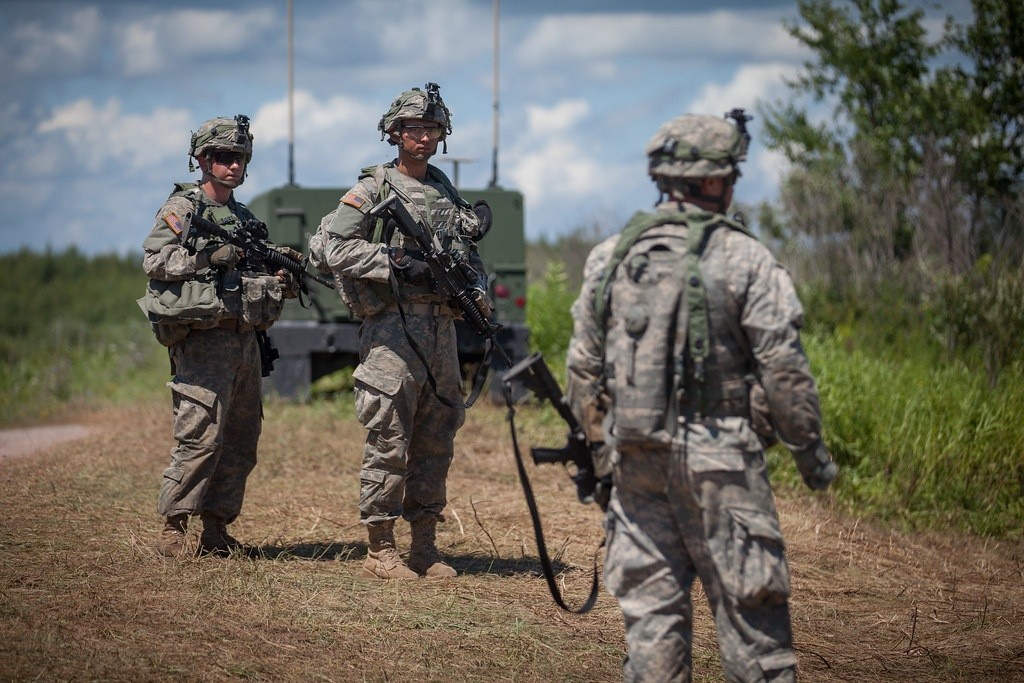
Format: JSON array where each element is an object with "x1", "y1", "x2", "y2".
[
  {"x1": 399, "y1": 257, "x2": 435, "y2": 287},
  {"x1": 795, "y1": 440, "x2": 840, "y2": 491}
]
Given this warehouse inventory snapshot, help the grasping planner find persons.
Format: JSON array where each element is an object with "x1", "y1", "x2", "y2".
[
  {"x1": 563, "y1": 115, "x2": 839, "y2": 683},
  {"x1": 325, "y1": 90, "x2": 495, "y2": 579},
  {"x1": 144, "y1": 117, "x2": 303, "y2": 559}
]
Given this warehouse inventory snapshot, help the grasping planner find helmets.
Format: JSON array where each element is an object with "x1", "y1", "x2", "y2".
[
  {"x1": 376, "y1": 88, "x2": 451, "y2": 141},
  {"x1": 645, "y1": 109, "x2": 749, "y2": 178},
  {"x1": 189, "y1": 117, "x2": 253, "y2": 157}
]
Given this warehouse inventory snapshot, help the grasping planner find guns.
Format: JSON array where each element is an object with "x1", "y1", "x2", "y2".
[
  {"x1": 502, "y1": 349, "x2": 614, "y2": 614},
  {"x1": 369, "y1": 195, "x2": 514, "y2": 373},
  {"x1": 181, "y1": 211, "x2": 334, "y2": 307}
]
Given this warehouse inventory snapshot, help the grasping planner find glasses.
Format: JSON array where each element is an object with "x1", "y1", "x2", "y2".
[
  {"x1": 728, "y1": 170, "x2": 742, "y2": 184},
  {"x1": 401, "y1": 124, "x2": 443, "y2": 138},
  {"x1": 209, "y1": 152, "x2": 247, "y2": 165}
]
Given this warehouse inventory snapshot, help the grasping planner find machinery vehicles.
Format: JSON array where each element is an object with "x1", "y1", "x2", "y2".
[{"x1": 242, "y1": 0, "x2": 534, "y2": 407}]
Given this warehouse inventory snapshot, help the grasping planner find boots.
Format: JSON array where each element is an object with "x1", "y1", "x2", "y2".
[
  {"x1": 199, "y1": 512, "x2": 241, "y2": 555},
  {"x1": 159, "y1": 510, "x2": 185, "y2": 559},
  {"x1": 409, "y1": 518, "x2": 460, "y2": 580},
  {"x1": 363, "y1": 521, "x2": 417, "y2": 581}
]
